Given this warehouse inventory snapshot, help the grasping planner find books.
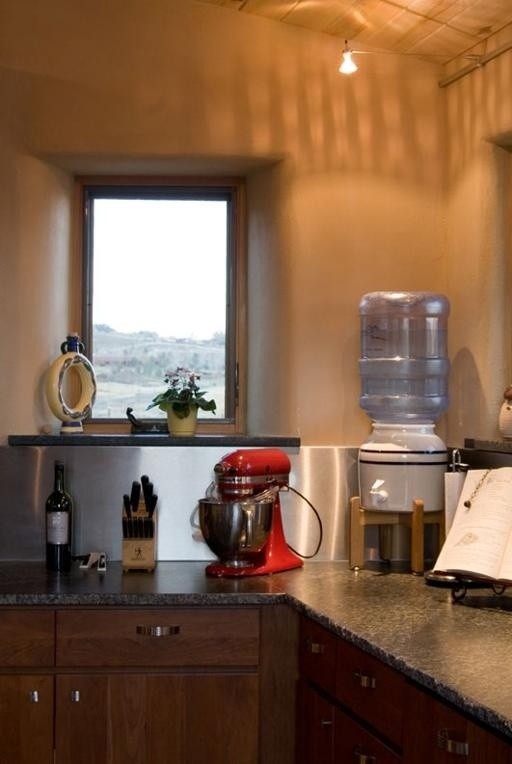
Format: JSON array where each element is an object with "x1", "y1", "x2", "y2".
[{"x1": 426, "y1": 467, "x2": 511, "y2": 587}]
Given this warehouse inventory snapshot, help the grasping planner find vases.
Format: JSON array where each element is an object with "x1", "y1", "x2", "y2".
[{"x1": 163, "y1": 399, "x2": 198, "y2": 437}]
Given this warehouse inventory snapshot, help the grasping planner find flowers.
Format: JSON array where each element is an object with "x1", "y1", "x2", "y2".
[{"x1": 162, "y1": 364, "x2": 200, "y2": 393}]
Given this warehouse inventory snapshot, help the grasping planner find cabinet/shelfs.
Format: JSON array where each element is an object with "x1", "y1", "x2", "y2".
[{"x1": 0, "y1": 602, "x2": 512, "y2": 763}]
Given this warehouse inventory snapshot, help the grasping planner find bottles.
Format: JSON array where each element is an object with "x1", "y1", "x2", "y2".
[{"x1": 45, "y1": 459, "x2": 73, "y2": 578}]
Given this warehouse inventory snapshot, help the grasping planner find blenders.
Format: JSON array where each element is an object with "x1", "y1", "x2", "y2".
[{"x1": 198, "y1": 448, "x2": 304, "y2": 577}]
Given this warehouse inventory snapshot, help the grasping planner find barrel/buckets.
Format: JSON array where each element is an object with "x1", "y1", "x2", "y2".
[{"x1": 356, "y1": 292, "x2": 451, "y2": 424}]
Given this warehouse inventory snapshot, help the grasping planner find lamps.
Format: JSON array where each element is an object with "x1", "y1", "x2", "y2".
[{"x1": 339, "y1": 40, "x2": 483, "y2": 75}]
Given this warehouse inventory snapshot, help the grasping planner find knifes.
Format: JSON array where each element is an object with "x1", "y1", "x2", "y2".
[{"x1": 121, "y1": 474, "x2": 158, "y2": 539}]
